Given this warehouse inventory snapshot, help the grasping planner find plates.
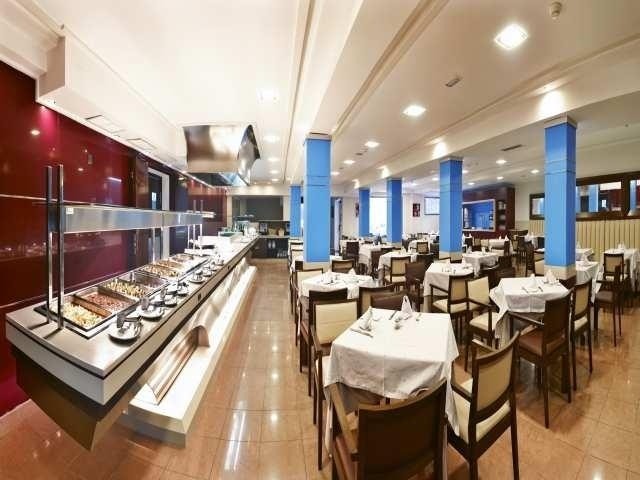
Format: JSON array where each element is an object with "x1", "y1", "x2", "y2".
[
  {"x1": 109, "y1": 322, "x2": 141, "y2": 340},
  {"x1": 136, "y1": 257, "x2": 224, "y2": 318}
]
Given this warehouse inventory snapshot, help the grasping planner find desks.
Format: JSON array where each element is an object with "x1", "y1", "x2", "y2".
[{"x1": 252, "y1": 235, "x2": 292, "y2": 258}]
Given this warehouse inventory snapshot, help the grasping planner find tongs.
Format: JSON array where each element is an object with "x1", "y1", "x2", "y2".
[{"x1": 116, "y1": 312, "x2": 143, "y2": 327}]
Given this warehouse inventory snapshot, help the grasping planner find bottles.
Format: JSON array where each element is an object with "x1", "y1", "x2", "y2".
[{"x1": 488, "y1": 209, "x2": 493, "y2": 229}]
